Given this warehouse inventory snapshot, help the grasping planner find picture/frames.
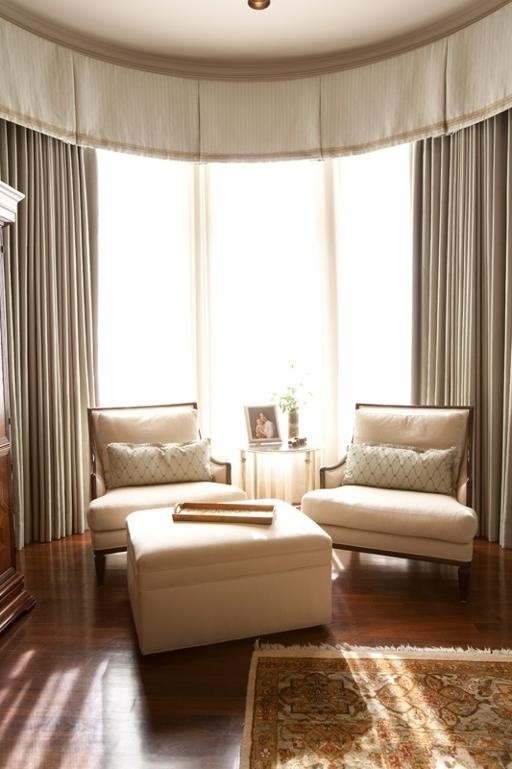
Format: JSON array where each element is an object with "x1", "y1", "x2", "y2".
[{"x1": 244, "y1": 404, "x2": 282, "y2": 444}]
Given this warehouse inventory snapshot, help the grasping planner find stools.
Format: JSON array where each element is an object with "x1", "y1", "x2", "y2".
[{"x1": 125, "y1": 496, "x2": 333, "y2": 655}]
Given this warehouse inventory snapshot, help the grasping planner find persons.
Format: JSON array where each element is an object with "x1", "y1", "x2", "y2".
[
  {"x1": 257, "y1": 411, "x2": 276, "y2": 438},
  {"x1": 254, "y1": 418, "x2": 264, "y2": 438}
]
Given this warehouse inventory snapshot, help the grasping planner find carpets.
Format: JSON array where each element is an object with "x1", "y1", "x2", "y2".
[{"x1": 237, "y1": 638, "x2": 511, "y2": 769}]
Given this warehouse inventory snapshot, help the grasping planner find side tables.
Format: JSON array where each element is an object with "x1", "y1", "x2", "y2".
[{"x1": 240, "y1": 444, "x2": 321, "y2": 500}]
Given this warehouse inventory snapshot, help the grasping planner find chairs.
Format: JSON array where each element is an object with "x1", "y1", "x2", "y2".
[
  {"x1": 300, "y1": 402, "x2": 480, "y2": 607},
  {"x1": 87, "y1": 401, "x2": 248, "y2": 589}
]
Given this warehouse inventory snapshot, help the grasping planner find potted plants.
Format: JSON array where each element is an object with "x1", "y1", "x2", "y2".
[{"x1": 271, "y1": 386, "x2": 300, "y2": 442}]
